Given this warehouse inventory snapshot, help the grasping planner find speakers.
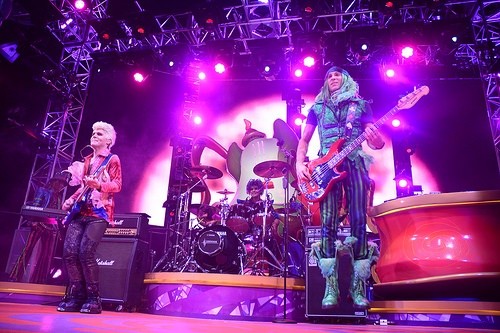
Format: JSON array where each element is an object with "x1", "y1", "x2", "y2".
[{"x1": 92, "y1": 236, "x2": 148, "y2": 307}]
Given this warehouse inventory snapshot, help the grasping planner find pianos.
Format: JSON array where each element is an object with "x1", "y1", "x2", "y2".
[{"x1": 20, "y1": 205, "x2": 71, "y2": 285}]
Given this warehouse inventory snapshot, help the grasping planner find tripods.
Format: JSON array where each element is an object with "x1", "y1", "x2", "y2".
[
  {"x1": 238, "y1": 180, "x2": 284, "y2": 277},
  {"x1": 152, "y1": 174, "x2": 201, "y2": 274}
]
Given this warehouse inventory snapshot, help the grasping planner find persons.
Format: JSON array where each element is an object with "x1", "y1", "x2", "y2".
[
  {"x1": 296, "y1": 66, "x2": 384, "y2": 310},
  {"x1": 234, "y1": 179, "x2": 282, "y2": 276},
  {"x1": 57, "y1": 121, "x2": 122, "y2": 314}
]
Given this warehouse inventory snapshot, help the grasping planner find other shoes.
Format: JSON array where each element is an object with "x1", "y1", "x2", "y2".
[
  {"x1": 56, "y1": 298, "x2": 87, "y2": 312},
  {"x1": 80, "y1": 294, "x2": 102, "y2": 314}
]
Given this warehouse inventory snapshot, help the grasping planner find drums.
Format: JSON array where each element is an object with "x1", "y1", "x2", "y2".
[
  {"x1": 192, "y1": 224, "x2": 247, "y2": 274},
  {"x1": 274, "y1": 214, "x2": 311, "y2": 243},
  {"x1": 197, "y1": 204, "x2": 221, "y2": 227},
  {"x1": 225, "y1": 204, "x2": 254, "y2": 233},
  {"x1": 251, "y1": 200, "x2": 275, "y2": 227}
]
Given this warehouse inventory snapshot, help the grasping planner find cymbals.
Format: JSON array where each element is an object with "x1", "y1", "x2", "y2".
[
  {"x1": 217, "y1": 191, "x2": 235, "y2": 194},
  {"x1": 253, "y1": 161, "x2": 291, "y2": 178},
  {"x1": 174, "y1": 183, "x2": 207, "y2": 192},
  {"x1": 190, "y1": 166, "x2": 224, "y2": 179},
  {"x1": 188, "y1": 204, "x2": 201, "y2": 217},
  {"x1": 274, "y1": 207, "x2": 297, "y2": 214}
]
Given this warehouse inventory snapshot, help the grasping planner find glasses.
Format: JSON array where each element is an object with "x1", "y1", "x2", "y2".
[{"x1": 92, "y1": 132, "x2": 103, "y2": 136}]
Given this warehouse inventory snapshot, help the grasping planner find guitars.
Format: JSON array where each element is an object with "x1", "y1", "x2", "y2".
[
  {"x1": 62, "y1": 164, "x2": 108, "y2": 225},
  {"x1": 297, "y1": 85, "x2": 430, "y2": 203}
]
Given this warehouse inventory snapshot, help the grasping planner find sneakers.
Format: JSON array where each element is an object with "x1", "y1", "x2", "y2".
[
  {"x1": 347, "y1": 273, "x2": 370, "y2": 308},
  {"x1": 322, "y1": 276, "x2": 341, "y2": 310}
]
